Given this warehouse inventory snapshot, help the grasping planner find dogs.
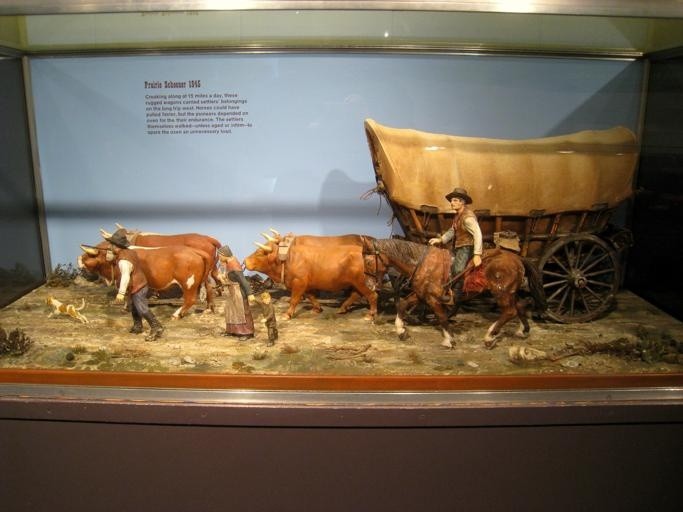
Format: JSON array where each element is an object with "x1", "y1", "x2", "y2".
[{"x1": 43, "y1": 294, "x2": 89, "y2": 326}]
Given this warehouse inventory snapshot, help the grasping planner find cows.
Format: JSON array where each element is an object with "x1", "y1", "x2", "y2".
[
  {"x1": 97, "y1": 222, "x2": 224, "y2": 299},
  {"x1": 76, "y1": 244, "x2": 216, "y2": 322},
  {"x1": 243, "y1": 232, "x2": 380, "y2": 321},
  {"x1": 269, "y1": 229, "x2": 375, "y2": 299}
]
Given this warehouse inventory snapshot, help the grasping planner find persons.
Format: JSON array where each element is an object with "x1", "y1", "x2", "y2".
[
  {"x1": 107, "y1": 228, "x2": 163, "y2": 342},
  {"x1": 213, "y1": 246, "x2": 254, "y2": 342},
  {"x1": 427, "y1": 188, "x2": 485, "y2": 303},
  {"x1": 249, "y1": 290, "x2": 278, "y2": 347}
]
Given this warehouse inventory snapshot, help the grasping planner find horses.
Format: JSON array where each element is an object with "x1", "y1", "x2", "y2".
[{"x1": 359, "y1": 234, "x2": 548, "y2": 349}]
